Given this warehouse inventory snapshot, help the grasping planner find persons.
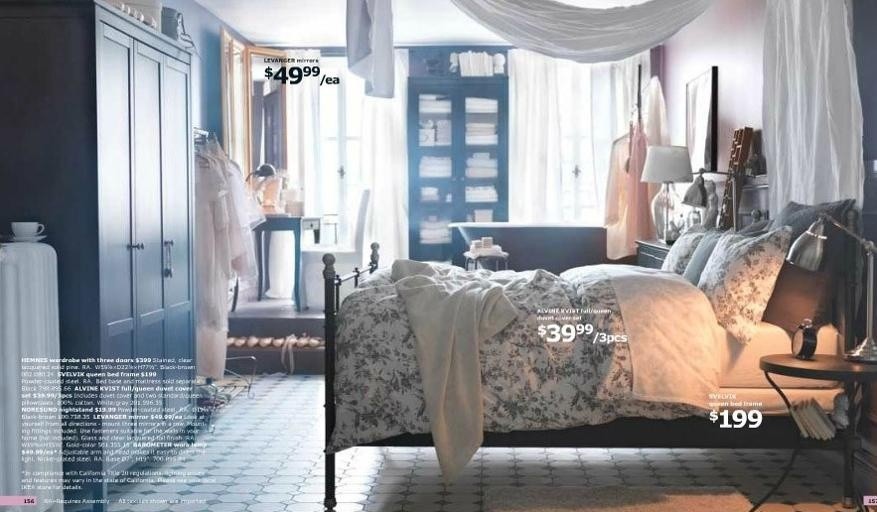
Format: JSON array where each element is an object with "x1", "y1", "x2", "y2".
[{"x1": 703, "y1": 180, "x2": 718, "y2": 227}]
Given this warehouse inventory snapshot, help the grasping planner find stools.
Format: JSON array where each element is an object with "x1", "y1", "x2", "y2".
[{"x1": 458, "y1": 243, "x2": 513, "y2": 271}]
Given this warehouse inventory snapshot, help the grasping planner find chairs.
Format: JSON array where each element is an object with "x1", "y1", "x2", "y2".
[{"x1": 295, "y1": 188, "x2": 373, "y2": 310}]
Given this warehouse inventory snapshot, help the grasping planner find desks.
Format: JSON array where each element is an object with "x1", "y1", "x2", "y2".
[
  {"x1": 747, "y1": 352, "x2": 877, "y2": 512},
  {"x1": 320, "y1": 201, "x2": 876, "y2": 512},
  {"x1": 229, "y1": 214, "x2": 338, "y2": 316}
]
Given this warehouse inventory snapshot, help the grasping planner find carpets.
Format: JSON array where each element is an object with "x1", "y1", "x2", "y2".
[{"x1": 479, "y1": 485, "x2": 751, "y2": 511}]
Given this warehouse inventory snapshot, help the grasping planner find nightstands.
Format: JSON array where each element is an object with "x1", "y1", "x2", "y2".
[{"x1": 632, "y1": 238, "x2": 672, "y2": 270}]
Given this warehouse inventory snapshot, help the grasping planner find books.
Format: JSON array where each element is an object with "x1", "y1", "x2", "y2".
[
  {"x1": 787, "y1": 394, "x2": 838, "y2": 442},
  {"x1": 416, "y1": 51, "x2": 496, "y2": 245}
]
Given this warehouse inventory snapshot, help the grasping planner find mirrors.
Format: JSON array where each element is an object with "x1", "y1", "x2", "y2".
[
  {"x1": 243, "y1": 45, "x2": 288, "y2": 176},
  {"x1": 681, "y1": 66, "x2": 716, "y2": 175},
  {"x1": 212, "y1": 29, "x2": 244, "y2": 175}
]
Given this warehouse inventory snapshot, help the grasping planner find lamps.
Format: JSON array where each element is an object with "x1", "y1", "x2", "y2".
[
  {"x1": 245, "y1": 163, "x2": 275, "y2": 213},
  {"x1": 682, "y1": 169, "x2": 739, "y2": 231},
  {"x1": 640, "y1": 144, "x2": 693, "y2": 239},
  {"x1": 784, "y1": 212, "x2": 876, "y2": 362}
]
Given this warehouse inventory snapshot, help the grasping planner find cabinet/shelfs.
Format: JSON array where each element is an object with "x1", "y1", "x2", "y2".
[
  {"x1": 0, "y1": 0, "x2": 194, "y2": 511},
  {"x1": 407, "y1": 48, "x2": 509, "y2": 259}
]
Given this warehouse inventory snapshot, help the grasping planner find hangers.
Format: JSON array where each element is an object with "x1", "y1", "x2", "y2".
[{"x1": 609, "y1": 104, "x2": 655, "y2": 144}]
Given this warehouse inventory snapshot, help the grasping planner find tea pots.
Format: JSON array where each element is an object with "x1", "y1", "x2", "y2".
[{"x1": 245, "y1": 160, "x2": 285, "y2": 216}]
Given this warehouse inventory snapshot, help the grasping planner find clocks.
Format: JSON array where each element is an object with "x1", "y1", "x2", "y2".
[{"x1": 788, "y1": 317, "x2": 819, "y2": 361}]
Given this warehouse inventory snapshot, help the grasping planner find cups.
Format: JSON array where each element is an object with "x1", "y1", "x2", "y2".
[
  {"x1": 9, "y1": 220, "x2": 48, "y2": 237},
  {"x1": 481, "y1": 236, "x2": 493, "y2": 249},
  {"x1": 471, "y1": 240, "x2": 482, "y2": 249}
]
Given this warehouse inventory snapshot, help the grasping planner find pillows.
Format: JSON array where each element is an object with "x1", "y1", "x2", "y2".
[
  {"x1": 677, "y1": 223, "x2": 762, "y2": 287},
  {"x1": 659, "y1": 230, "x2": 696, "y2": 277},
  {"x1": 698, "y1": 226, "x2": 793, "y2": 342}
]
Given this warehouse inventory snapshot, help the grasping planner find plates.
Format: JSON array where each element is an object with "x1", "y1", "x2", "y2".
[{"x1": 6, "y1": 236, "x2": 49, "y2": 245}]
